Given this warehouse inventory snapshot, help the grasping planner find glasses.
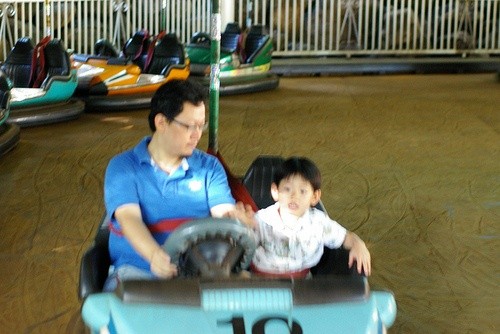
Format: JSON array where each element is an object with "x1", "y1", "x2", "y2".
[{"x1": 171, "y1": 119, "x2": 208, "y2": 133}]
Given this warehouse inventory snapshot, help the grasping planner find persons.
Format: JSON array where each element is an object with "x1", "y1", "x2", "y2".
[
  {"x1": 103, "y1": 78, "x2": 258, "y2": 294},
  {"x1": 249, "y1": 156, "x2": 371, "y2": 280}
]
